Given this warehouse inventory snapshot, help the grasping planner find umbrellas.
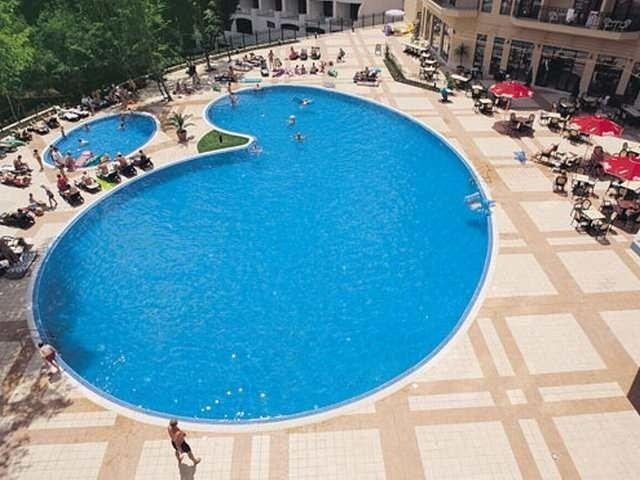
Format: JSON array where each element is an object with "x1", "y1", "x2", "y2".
[{"x1": 385, "y1": 8, "x2": 405, "y2": 27}]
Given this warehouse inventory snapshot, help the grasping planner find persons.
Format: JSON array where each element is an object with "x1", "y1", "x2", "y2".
[
  {"x1": 0, "y1": 79, "x2": 149, "y2": 265},
  {"x1": 492, "y1": 64, "x2": 639, "y2": 228},
  {"x1": 38, "y1": 341, "x2": 60, "y2": 373},
  {"x1": 439, "y1": 85, "x2": 448, "y2": 103},
  {"x1": 172, "y1": 43, "x2": 376, "y2": 154},
  {"x1": 167, "y1": 417, "x2": 201, "y2": 464}
]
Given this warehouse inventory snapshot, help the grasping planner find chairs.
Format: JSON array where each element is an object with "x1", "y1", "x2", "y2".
[
  {"x1": 451, "y1": 68, "x2": 640, "y2": 239},
  {"x1": 353, "y1": 11, "x2": 443, "y2": 87},
  {"x1": 0, "y1": 79, "x2": 153, "y2": 279},
  {"x1": 168, "y1": 52, "x2": 263, "y2": 100}
]
